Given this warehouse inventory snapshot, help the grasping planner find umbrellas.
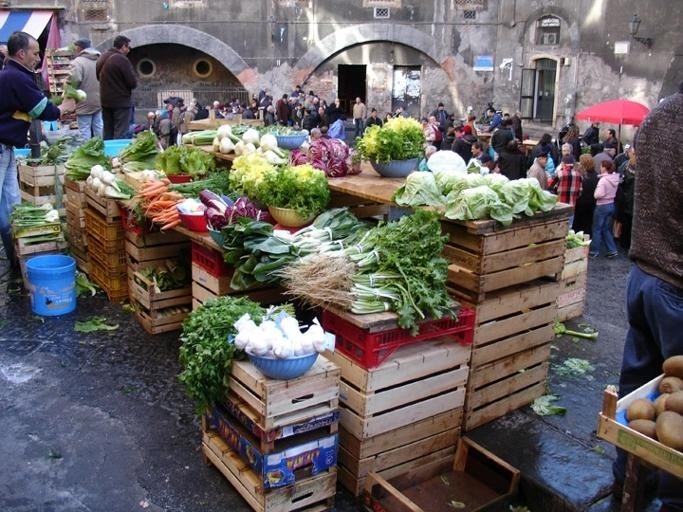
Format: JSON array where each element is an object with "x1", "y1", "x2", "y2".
[{"x1": 576, "y1": 97, "x2": 652, "y2": 154}]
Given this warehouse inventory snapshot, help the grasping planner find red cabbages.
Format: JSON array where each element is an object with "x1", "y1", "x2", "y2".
[
  {"x1": 199, "y1": 188, "x2": 269, "y2": 231},
  {"x1": 288, "y1": 137, "x2": 363, "y2": 177}
]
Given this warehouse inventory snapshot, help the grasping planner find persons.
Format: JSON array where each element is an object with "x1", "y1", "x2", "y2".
[
  {"x1": 421, "y1": 103, "x2": 636, "y2": 256},
  {"x1": 0, "y1": 30, "x2": 76, "y2": 261},
  {"x1": 611, "y1": 79, "x2": 683, "y2": 499},
  {"x1": 0, "y1": 44, "x2": 7, "y2": 70},
  {"x1": 147, "y1": 86, "x2": 346, "y2": 143},
  {"x1": 65, "y1": 38, "x2": 104, "y2": 143},
  {"x1": 353, "y1": 96, "x2": 409, "y2": 140},
  {"x1": 95, "y1": 35, "x2": 139, "y2": 140}
]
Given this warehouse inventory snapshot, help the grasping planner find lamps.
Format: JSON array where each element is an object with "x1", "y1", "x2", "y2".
[{"x1": 627, "y1": 11, "x2": 653, "y2": 48}]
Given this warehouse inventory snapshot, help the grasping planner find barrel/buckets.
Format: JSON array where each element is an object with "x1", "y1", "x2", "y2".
[{"x1": 25, "y1": 254, "x2": 76, "y2": 316}]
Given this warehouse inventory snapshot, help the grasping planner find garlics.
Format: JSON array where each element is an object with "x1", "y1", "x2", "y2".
[
  {"x1": 233, "y1": 313, "x2": 326, "y2": 360},
  {"x1": 212, "y1": 124, "x2": 286, "y2": 159}
]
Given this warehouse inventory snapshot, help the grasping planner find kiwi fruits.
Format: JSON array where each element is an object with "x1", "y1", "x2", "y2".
[{"x1": 625, "y1": 356, "x2": 683, "y2": 448}]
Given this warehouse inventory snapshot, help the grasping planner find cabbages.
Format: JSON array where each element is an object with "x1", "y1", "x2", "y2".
[{"x1": 391, "y1": 150, "x2": 559, "y2": 227}]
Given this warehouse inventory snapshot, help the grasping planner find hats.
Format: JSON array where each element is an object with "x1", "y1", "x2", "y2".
[{"x1": 73, "y1": 38, "x2": 91, "y2": 48}]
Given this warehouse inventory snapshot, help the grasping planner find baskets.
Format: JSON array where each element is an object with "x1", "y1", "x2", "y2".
[
  {"x1": 273, "y1": 129, "x2": 308, "y2": 149},
  {"x1": 368, "y1": 157, "x2": 420, "y2": 179},
  {"x1": 244, "y1": 352, "x2": 321, "y2": 381},
  {"x1": 267, "y1": 202, "x2": 317, "y2": 230}
]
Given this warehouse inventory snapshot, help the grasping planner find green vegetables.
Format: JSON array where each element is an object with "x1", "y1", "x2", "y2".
[
  {"x1": 174, "y1": 295, "x2": 296, "y2": 415},
  {"x1": 183, "y1": 125, "x2": 290, "y2": 145}
]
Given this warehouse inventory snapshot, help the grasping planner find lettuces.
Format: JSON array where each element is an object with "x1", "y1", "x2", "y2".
[{"x1": 64, "y1": 131, "x2": 215, "y2": 180}]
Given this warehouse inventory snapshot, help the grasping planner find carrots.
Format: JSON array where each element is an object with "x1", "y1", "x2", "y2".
[{"x1": 124, "y1": 178, "x2": 185, "y2": 235}]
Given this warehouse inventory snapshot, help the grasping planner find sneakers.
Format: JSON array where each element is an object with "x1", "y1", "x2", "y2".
[{"x1": 588, "y1": 251, "x2": 618, "y2": 260}]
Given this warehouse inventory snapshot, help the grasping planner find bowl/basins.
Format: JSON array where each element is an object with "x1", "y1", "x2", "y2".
[
  {"x1": 276, "y1": 135, "x2": 307, "y2": 149},
  {"x1": 177, "y1": 213, "x2": 212, "y2": 234},
  {"x1": 370, "y1": 158, "x2": 419, "y2": 176},
  {"x1": 167, "y1": 175, "x2": 192, "y2": 184},
  {"x1": 102, "y1": 138, "x2": 132, "y2": 160},
  {"x1": 244, "y1": 348, "x2": 320, "y2": 380},
  {"x1": 12, "y1": 148, "x2": 33, "y2": 157}
]
